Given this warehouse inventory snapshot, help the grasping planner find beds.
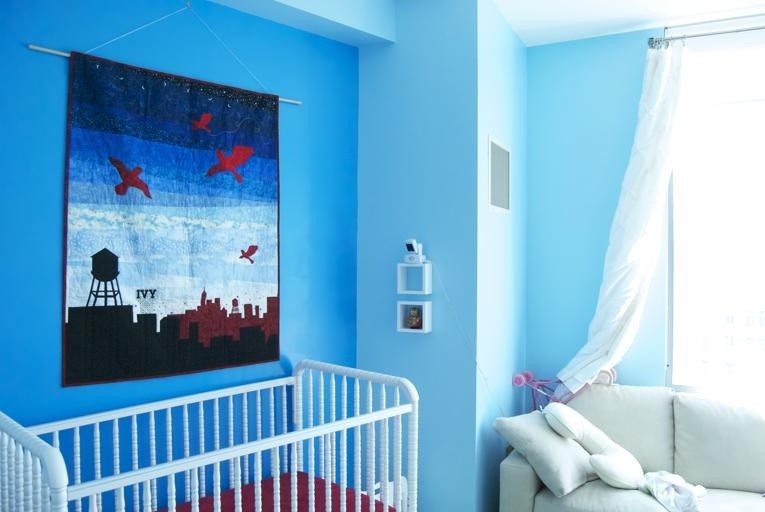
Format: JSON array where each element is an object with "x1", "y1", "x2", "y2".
[{"x1": 0, "y1": 359, "x2": 420, "y2": 511}]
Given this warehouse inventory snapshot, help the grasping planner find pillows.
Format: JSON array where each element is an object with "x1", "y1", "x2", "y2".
[{"x1": 491, "y1": 403, "x2": 645, "y2": 497}]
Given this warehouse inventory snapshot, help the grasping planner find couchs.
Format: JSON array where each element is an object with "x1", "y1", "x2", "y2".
[{"x1": 500, "y1": 381, "x2": 765, "y2": 511}]
